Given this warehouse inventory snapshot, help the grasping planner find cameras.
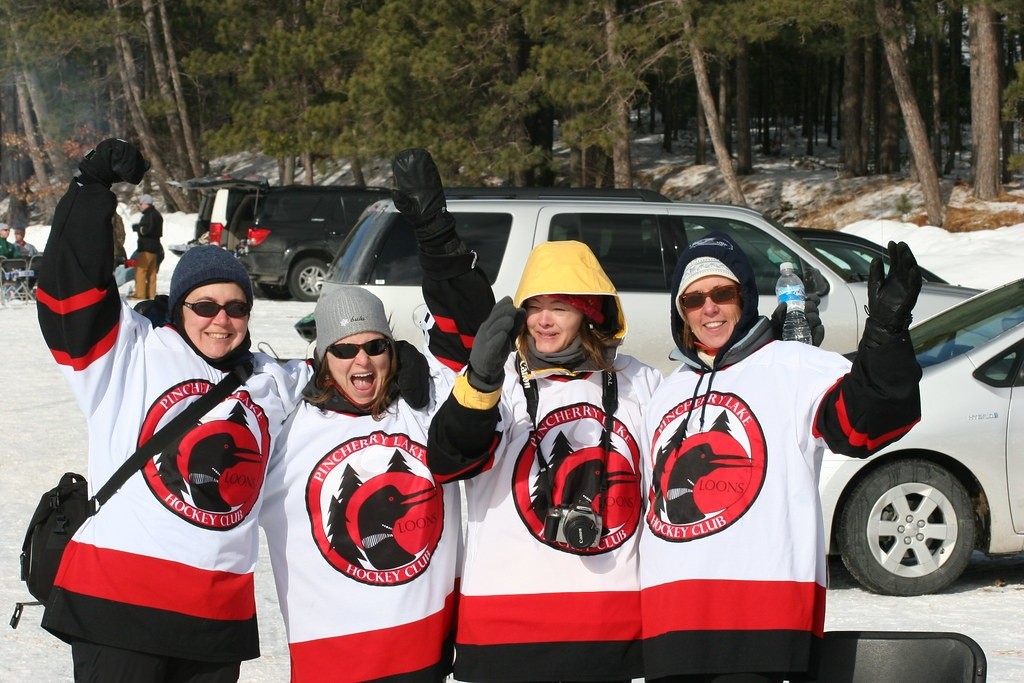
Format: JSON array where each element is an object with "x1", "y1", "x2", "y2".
[{"x1": 544, "y1": 505, "x2": 603, "y2": 549}]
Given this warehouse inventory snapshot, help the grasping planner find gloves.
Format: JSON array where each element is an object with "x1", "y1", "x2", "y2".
[
  {"x1": 466, "y1": 295, "x2": 527, "y2": 393},
  {"x1": 392, "y1": 148, "x2": 456, "y2": 242},
  {"x1": 398, "y1": 344, "x2": 429, "y2": 409},
  {"x1": 858, "y1": 239, "x2": 922, "y2": 359},
  {"x1": 132, "y1": 224, "x2": 141, "y2": 232},
  {"x1": 72, "y1": 138, "x2": 150, "y2": 187}
]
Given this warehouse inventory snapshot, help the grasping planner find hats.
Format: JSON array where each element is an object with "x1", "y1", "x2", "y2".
[
  {"x1": 314, "y1": 286, "x2": 393, "y2": 363},
  {"x1": 673, "y1": 257, "x2": 741, "y2": 323},
  {"x1": 538, "y1": 293, "x2": 604, "y2": 324},
  {"x1": 170, "y1": 247, "x2": 253, "y2": 327},
  {"x1": 140, "y1": 193, "x2": 153, "y2": 204},
  {"x1": 0, "y1": 222, "x2": 10, "y2": 231}
]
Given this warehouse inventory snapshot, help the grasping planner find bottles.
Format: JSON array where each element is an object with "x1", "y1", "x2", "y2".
[{"x1": 775, "y1": 262, "x2": 812, "y2": 346}]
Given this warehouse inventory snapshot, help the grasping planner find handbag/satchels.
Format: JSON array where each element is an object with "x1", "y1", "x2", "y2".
[{"x1": 21, "y1": 472, "x2": 96, "y2": 609}]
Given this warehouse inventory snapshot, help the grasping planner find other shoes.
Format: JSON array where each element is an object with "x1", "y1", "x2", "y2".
[{"x1": 127, "y1": 295, "x2": 139, "y2": 300}]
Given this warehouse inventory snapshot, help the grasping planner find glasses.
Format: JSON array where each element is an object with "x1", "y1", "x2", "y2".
[
  {"x1": 326, "y1": 338, "x2": 390, "y2": 360},
  {"x1": 3, "y1": 228, "x2": 10, "y2": 232},
  {"x1": 678, "y1": 284, "x2": 742, "y2": 311},
  {"x1": 15, "y1": 231, "x2": 22, "y2": 235},
  {"x1": 183, "y1": 300, "x2": 251, "y2": 318}
]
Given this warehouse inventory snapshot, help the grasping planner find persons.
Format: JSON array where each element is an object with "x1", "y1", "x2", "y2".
[
  {"x1": 35, "y1": 138, "x2": 431, "y2": 683},
  {"x1": 111, "y1": 214, "x2": 127, "y2": 260},
  {"x1": 0, "y1": 223, "x2": 38, "y2": 300},
  {"x1": 260, "y1": 148, "x2": 497, "y2": 683},
  {"x1": 131, "y1": 195, "x2": 162, "y2": 299},
  {"x1": 642, "y1": 233, "x2": 921, "y2": 683},
  {"x1": 426, "y1": 239, "x2": 826, "y2": 683}
]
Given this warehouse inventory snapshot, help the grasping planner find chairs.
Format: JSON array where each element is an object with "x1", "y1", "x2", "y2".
[{"x1": 0, "y1": 253, "x2": 44, "y2": 306}]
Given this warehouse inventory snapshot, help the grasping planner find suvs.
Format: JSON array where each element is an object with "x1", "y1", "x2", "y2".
[
  {"x1": 293, "y1": 188, "x2": 1024, "y2": 381},
  {"x1": 813, "y1": 277, "x2": 1023, "y2": 597},
  {"x1": 166, "y1": 173, "x2": 395, "y2": 302}
]
